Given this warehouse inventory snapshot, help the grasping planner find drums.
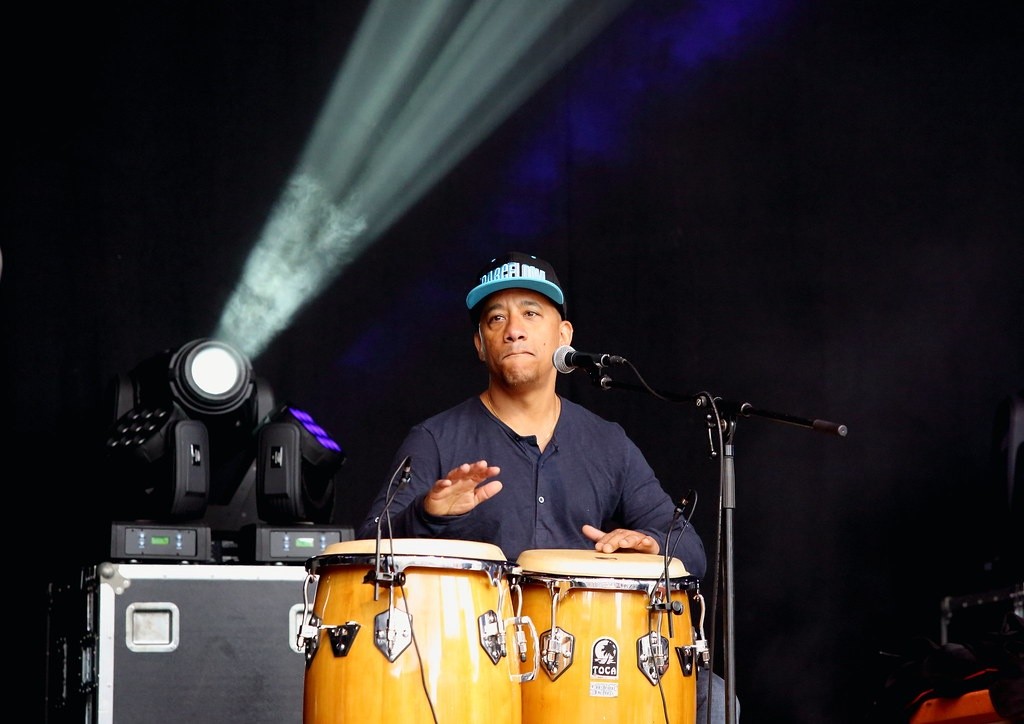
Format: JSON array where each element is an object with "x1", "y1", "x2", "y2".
[
  {"x1": 510, "y1": 549, "x2": 712, "y2": 724},
  {"x1": 297, "y1": 537, "x2": 541, "y2": 724}
]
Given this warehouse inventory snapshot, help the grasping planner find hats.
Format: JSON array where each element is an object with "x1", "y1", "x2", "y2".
[{"x1": 466, "y1": 253, "x2": 568, "y2": 320}]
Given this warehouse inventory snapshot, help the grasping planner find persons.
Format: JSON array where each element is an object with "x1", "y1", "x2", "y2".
[{"x1": 351, "y1": 248, "x2": 741, "y2": 724}]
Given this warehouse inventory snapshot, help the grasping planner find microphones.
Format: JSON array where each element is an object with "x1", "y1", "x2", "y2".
[{"x1": 553, "y1": 344, "x2": 629, "y2": 373}]
